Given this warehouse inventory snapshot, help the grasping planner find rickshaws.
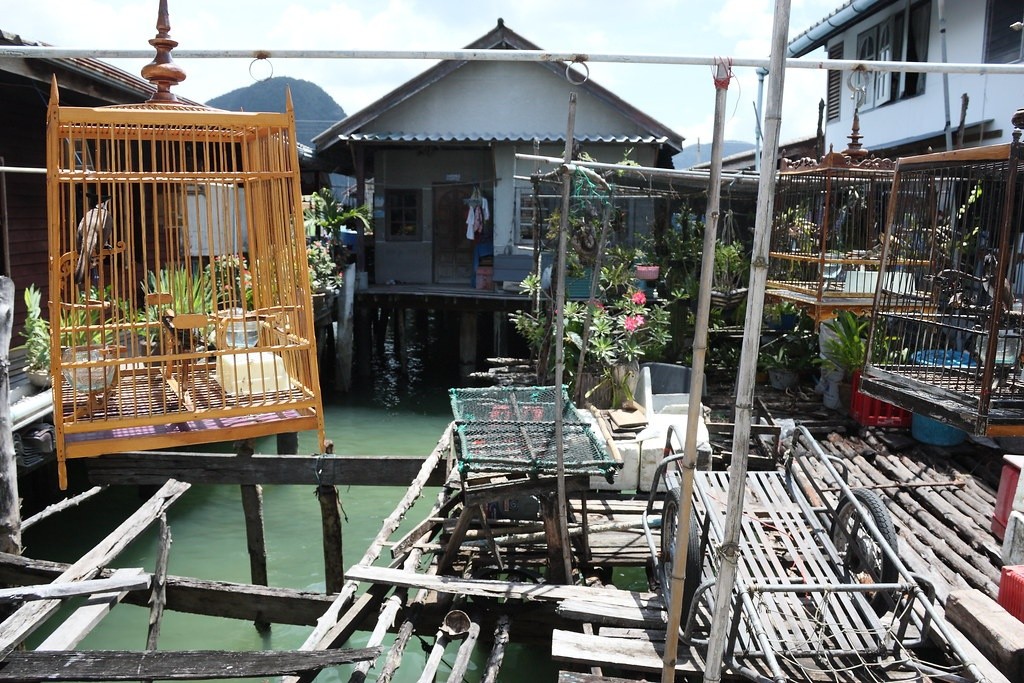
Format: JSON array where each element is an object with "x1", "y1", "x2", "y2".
[{"x1": 641, "y1": 422, "x2": 985, "y2": 682}]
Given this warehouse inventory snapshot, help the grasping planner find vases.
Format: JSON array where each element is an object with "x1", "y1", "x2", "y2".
[{"x1": 573, "y1": 370, "x2": 617, "y2": 409}]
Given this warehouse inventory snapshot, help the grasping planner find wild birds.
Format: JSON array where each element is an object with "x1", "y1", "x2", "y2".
[
  {"x1": 74, "y1": 193, "x2": 113, "y2": 286},
  {"x1": 746, "y1": 198, "x2": 1016, "y2": 368}
]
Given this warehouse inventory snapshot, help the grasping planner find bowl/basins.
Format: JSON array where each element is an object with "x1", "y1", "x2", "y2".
[
  {"x1": 219, "y1": 308, "x2": 264, "y2": 347},
  {"x1": 818, "y1": 253, "x2": 842, "y2": 278},
  {"x1": 63, "y1": 345, "x2": 115, "y2": 390},
  {"x1": 977, "y1": 329, "x2": 1022, "y2": 365}
]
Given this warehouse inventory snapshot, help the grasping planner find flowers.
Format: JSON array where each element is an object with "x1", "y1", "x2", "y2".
[{"x1": 623, "y1": 291, "x2": 646, "y2": 333}]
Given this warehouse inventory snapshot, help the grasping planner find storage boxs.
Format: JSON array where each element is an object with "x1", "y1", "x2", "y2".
[
  {"x1": 475, "y1": 265, "x2": 493, "y2": 289},
  {"x1": 851, "y1": 370, "x2": 912, "y2": 428}
]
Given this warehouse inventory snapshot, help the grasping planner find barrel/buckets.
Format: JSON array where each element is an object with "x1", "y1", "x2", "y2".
[
  {"x1": 819, "y1": 319, "x2": 883, "y2": 409},
  {"x1": 912, "y1": 350, "x2": 978, "y2": 445}
]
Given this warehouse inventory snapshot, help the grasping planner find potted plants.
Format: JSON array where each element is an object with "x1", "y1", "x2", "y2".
[
  {"x1": 120, "y1": 265, "x2": 227, "y2": 368},
  {"x1": 810, "y1": 308, "x2": 909, "y2": 415},
  {"x1": 9, "y1": 284, "x2": 115, "y2": 388}
]
